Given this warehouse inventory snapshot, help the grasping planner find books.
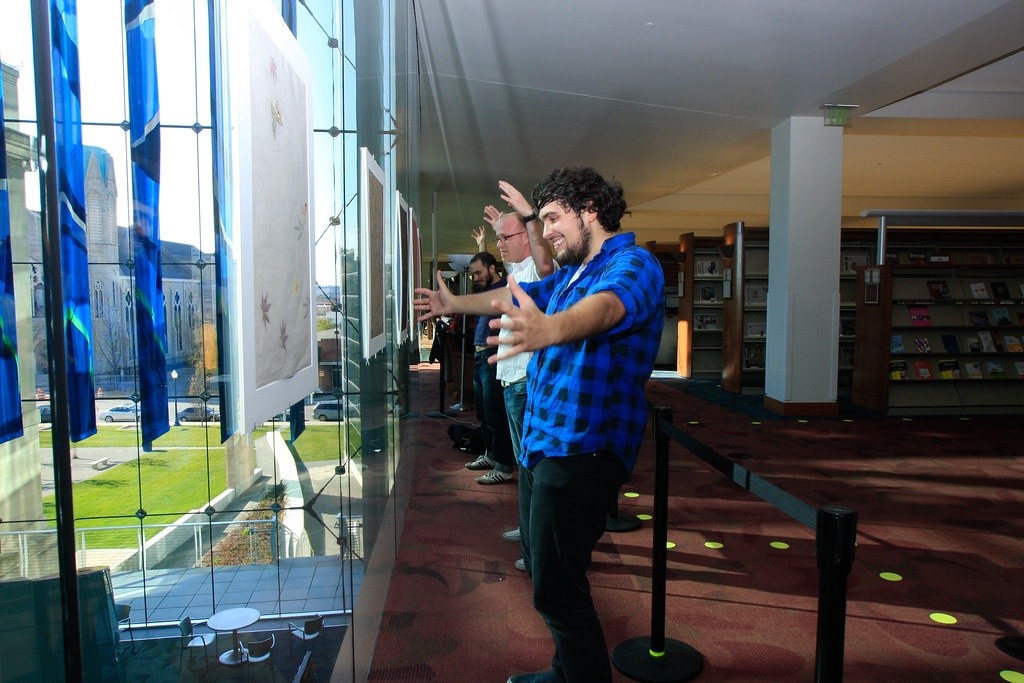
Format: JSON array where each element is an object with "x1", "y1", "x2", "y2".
[{"x1": 885, "y1": 251, "x2": 1024, "y2": 380}]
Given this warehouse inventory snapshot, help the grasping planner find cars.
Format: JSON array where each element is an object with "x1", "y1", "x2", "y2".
[
  {"x1": 313, "y1": 401, "x2": 358, "y2": 421},
  {"x1": 99, "y1": 403, "x2": 141, "y2": 423},
  {"x1": 177, "y1": 406, "x2": 220, "y2": 422}
]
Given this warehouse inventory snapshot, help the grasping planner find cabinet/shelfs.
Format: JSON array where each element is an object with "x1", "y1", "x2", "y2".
[
  {"x1": 644, "y1": 240, "x2": 677, "y2": 309},
  {"x1": 676, "y1": 231, "x2": 721, "y2": 380},
  {"x1": 850, "y1": 263, "x2": 1024, "y2": 417},
  {"x1": 720, "y1": 221, "x2": 1024, "y2": 394}
]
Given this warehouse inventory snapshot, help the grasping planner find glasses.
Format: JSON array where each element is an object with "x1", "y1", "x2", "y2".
[{"x1": 493, "y1": 229, "x2": 525, "y2": 242}]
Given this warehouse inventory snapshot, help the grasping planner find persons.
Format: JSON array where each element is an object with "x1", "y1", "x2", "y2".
[
  {"x1": 466, "y1": 224, "x2": 518, "y2": 484},
  {"x1": 482, "y1": 179, "x2": 561, "y2": 540},
  {"x1": 412, "y1": 164, "x2": 669, "y2": 683}
]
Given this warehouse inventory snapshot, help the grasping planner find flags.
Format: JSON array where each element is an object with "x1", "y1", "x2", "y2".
[
  {"x1": 0, "y1": 59, "x2": 26, "y2": 445},
  {"x1": 123, "y1": 0, "x2": 172, "y2": 455},
  {"x1": 48, "y1": 0, "x2": 97, "y2": 443}
]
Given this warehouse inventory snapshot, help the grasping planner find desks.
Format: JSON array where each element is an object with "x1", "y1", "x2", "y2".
[{"x1": 206, "y1": 607, "x2": 262, "y2": 666}]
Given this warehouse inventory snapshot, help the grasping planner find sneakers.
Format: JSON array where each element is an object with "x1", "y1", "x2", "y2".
[
  {"x1": 465, "y1": 455, "x2": 496, "y2": 468},
  {"x1": 475, "y1": 469, "x2": 514, "y2": 483}
]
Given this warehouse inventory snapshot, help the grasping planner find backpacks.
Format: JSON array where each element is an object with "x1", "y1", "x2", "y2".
[{"x1": 447, "y1": 426, "x2": 485, "y2": 456}]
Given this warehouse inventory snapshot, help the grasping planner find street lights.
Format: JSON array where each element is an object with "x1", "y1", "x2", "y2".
[{"x1": 171, "y1": 369, "x2": 182, "y2": 426}]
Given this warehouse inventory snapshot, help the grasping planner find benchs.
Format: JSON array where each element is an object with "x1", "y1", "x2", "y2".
[{"x1": 89, "y1": 457, "x2": 110, "y2": 470}]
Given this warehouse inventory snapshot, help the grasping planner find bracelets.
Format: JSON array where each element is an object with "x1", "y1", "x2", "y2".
[{"x1": 523, "y1": 212, "x2": 537, "y2": 222}]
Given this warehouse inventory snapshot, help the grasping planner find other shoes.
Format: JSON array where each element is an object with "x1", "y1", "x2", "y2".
[
  {"x1": 503, "y1": 526, "x2": 522, "y2": 541},
  {"x1": 507, "y1": 668, "x2": 563, "y2": 683},
  {"x1": 516, "y1": 558, "x2": 526, "y2": 569}
]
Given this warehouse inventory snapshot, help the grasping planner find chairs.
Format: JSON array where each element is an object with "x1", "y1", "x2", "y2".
[
  {"x1": 289, "y1": 614, "x2": 324, "y2": 660},
  {"x1": 238, "y1": 633, "x2": 276, "y2": 683},
  {"x1": 114, "y1": 604, "x2": 136, "y2": 650},
  {"x1": 176, "y1": 615, "x2": 219, "y2": 674}
]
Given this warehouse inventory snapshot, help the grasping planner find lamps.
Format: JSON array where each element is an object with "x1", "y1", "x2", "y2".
[
  {"x1": 677, "y1": 252, "x2": 686, "y2": 264},
  {"x1": 721, "y1": 245, "x2": 734, "y2": 259}
]
